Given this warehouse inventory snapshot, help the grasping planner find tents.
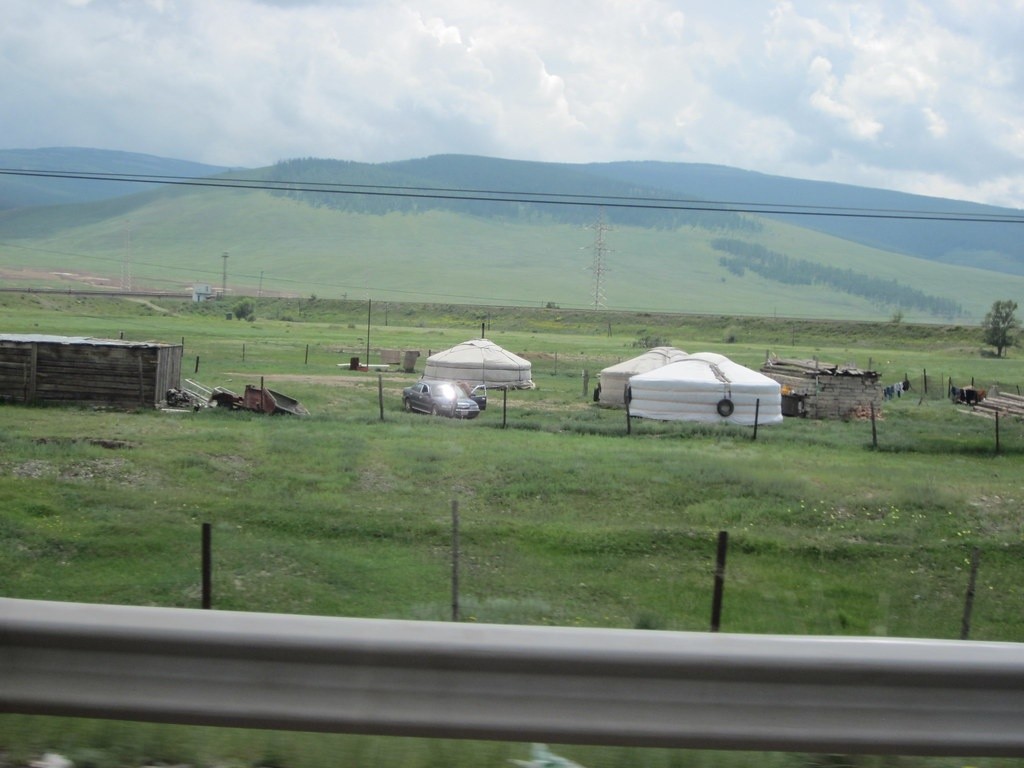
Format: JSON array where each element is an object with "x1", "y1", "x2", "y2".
[
  {"x1": 598, "y1": 346, "x2": 689, "y2": 409},
  {"x1": 423, "y1": 338, "x2": 536, "y2": 390},
  {"x1": 625, "y1": 352, "x2": 783, "y2": 425}
]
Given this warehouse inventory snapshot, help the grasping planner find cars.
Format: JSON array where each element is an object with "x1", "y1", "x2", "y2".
[{"x1": 402, "y1": 380, "x2": 487, "y2": 419}]
